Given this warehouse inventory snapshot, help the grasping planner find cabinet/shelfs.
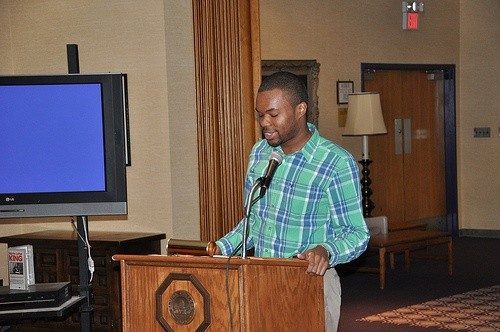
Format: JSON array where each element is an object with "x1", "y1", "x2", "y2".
[{"x1": 0, "y1": 229, "x2": 167, "y2": 332}]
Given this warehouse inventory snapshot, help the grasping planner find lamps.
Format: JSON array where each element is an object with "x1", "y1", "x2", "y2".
[
  {"x1": 342, "y1": 92, "x2": 388, "y2": 218},
  {"x1": 402, "y1": 1, "x2": 423, "y2": 13}
]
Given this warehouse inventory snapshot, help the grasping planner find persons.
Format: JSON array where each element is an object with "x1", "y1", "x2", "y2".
[{"x1": 207, "y1": 71, "x2": 370, "y2": 332}]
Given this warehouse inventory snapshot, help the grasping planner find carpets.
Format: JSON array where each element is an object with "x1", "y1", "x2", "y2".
[{"x1": 354, "y1": 284, "x2": 500, "y2": 332}]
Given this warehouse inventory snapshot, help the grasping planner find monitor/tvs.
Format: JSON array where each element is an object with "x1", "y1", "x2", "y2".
[{"x1": 0, "y1": 73, "x2": 127, "y2": 218}]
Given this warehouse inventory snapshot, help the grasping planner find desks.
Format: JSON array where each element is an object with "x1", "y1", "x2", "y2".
[{"x1": 363, "y1": 231, "x2": 454, "y2": 290}]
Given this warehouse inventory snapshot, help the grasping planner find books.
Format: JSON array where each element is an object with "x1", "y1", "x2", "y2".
[
  {"x1": 8, "y1": 245, "x2": 35, "y2": 290},
  {"x1": 166, "y1": 239, "x2": 209, "y2": 256}
]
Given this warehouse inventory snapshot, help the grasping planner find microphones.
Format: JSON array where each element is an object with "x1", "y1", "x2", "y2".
[{"x1": 259, "y1": 152, "x2": 283, "y2": 198}]
